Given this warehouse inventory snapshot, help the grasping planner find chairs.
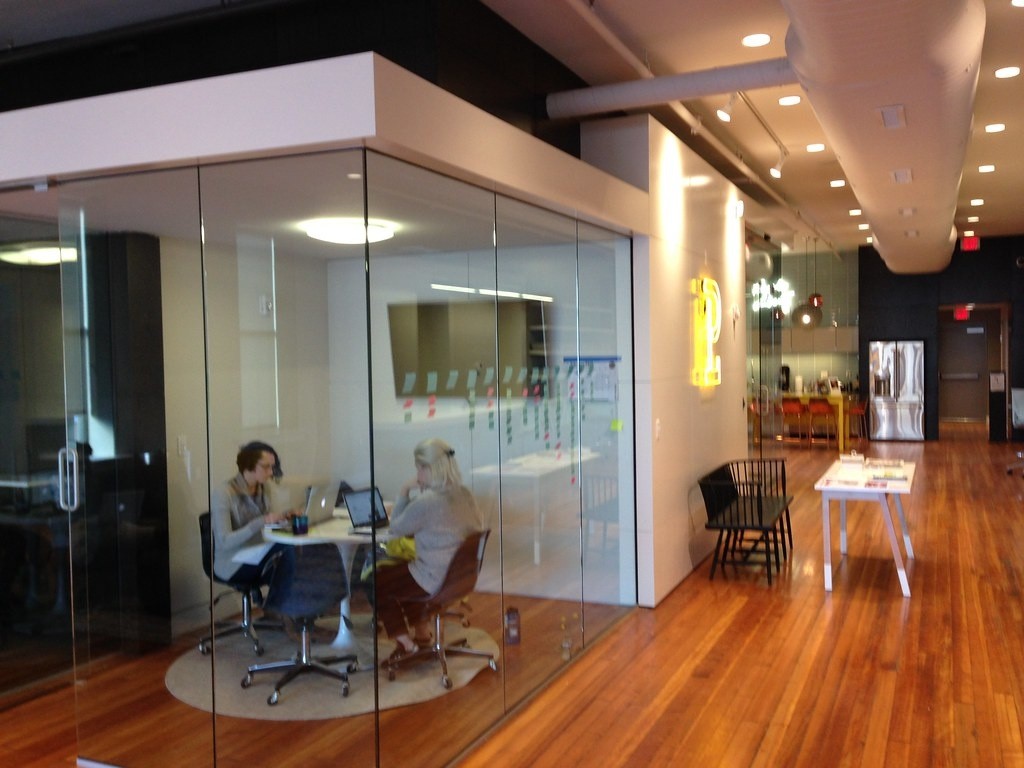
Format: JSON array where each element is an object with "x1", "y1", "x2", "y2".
[
  {"x1": 380, "y1": 529, "x2": 499, "y2": 689},
  {"x1": 698, "y1": 457, "x2": 795, "y2": 585},
  {"x1": 197, "y1": 509, "x2": 289, "y2": 656},
  {"x1": 239, "y1": 542, "x2": 358, "y2": 706},
  {"x1": 1006, "y1": 387, "x2": 1024, "y2": 478},
  {"x1": 578, "y1": 475, "x2": 619, "y2": 565}
]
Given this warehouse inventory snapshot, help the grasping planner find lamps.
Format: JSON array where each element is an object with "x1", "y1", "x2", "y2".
[{"x1": 792, "y1": 233, "x2": 824, "y2": 332}]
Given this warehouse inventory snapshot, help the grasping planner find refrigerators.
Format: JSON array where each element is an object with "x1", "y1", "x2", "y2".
[{"x1": 869, "y1": 340, "x2": 925, "y2": 441}]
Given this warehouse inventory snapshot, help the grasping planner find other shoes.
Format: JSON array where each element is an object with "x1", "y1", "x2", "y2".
[
  {"x1": 384, "y1": 645, "x2": 419, "y2": 668},
  {"x1": 284, "y1": 615, "x2": 302, "y2": 643},
  {"x1": 412, "y1": 632, "x2": 433, "y2": 647},
  {"x1": 308, "y1": 618, "x2": 324, "y2": 632}
]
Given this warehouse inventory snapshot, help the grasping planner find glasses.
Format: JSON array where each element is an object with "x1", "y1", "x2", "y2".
[{"x1": 258, "y1": 462, "x2": 276, "y2": 470}]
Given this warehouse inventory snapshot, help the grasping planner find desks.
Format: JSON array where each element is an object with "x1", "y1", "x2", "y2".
[
  {"x1": 813, "y1": 459, "x2": 918, "y2": 598},
  {"x1": 469, "y1": 444, "x2": 602, "y2": 564},
  {"x1": 261, "y1": 503, "x2": 413, "y2": 675},
  {"x1": 751, "y1": 393, "x2": 851, "y2": 455}
]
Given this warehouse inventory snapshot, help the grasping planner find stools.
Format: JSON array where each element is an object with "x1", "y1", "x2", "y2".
[
  {"x1": 749, "y1": 397, "x2": 838, "y2": 451},
  {"x1": 843, "y1": 397, "x2": 870, "y2": 448}
]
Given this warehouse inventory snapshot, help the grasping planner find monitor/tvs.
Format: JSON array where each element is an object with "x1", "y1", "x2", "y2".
[{"x1": 387, "y1": 298, "x2": 547, "y2": 398}]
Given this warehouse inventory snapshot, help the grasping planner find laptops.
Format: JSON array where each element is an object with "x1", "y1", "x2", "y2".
[
  {"x1": 343, "y1": 488, "x2": 389, "y2": 534},
  {"x1": 264, "y1": 479, "x2": 342, "y2": 529}
]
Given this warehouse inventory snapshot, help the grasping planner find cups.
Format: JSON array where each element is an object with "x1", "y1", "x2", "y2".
[
  {"x1": 795, "y1": 376, "x2": 803, "y2": 393},
  {"x1": 292, "y1": 514, "x2": 308, "y2": 535}
]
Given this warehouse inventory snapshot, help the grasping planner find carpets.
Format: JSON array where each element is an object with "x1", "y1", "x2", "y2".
[{"x1": 165, "y1": 614, "x2": 500, "y2": 721}]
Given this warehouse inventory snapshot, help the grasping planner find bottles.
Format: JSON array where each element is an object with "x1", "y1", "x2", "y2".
[{"x1": 504, "y1": 607, "x2": 521, "y2": 644}]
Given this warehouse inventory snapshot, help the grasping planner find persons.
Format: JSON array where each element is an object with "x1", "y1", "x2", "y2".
[
  {"x1": 375, "y1": 440, "x2": 484, "y2": 667},
  {"x1": 209, "y1": 441, "x2": 304, "y2": 644}
]
